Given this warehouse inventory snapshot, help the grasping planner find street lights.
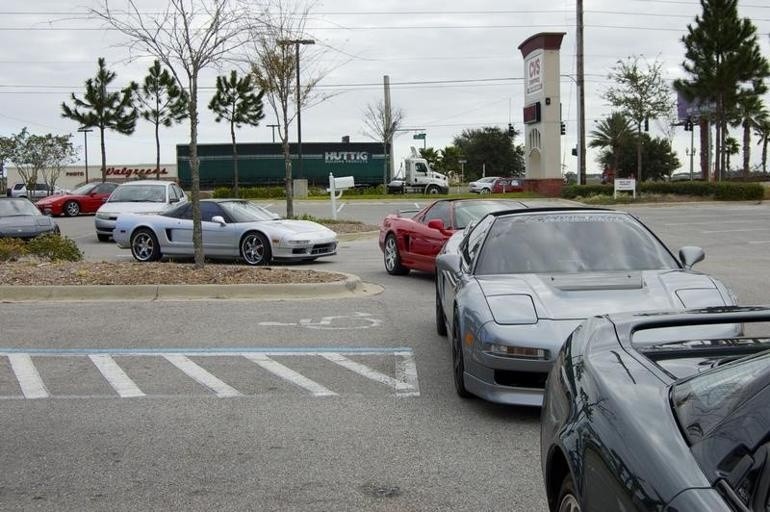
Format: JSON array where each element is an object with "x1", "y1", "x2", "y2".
[{"x1": 276, "y1": 38, "x2": 314, "y2": 180}]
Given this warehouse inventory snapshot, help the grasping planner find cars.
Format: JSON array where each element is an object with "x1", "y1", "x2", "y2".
[
  {"x1": 113, "y1": 199, "x2": 339, "y2": 266},
  {"x1": 0, "y1": 197, "x2": 60, "y2": 242},
  {"x1": 470, "y1": 176, "x2": 523, "y2": 194},
  {"x1": 94, "y1": 180, "x2": 188, "y2": 241},
  {"x1": 33, "y1": 181, "x2": 122, "y2": 216}
]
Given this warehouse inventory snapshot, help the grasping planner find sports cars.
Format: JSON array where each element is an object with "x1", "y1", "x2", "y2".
[
  {"x1": 436, "y1": 206, "x2": 744, "y2": 407},
  {"x1": 378, "y1": 197, "x2": 588, "y2": 273},
  {"x1": 542, "y1": 306, "x2": 769, "y2": 511}
]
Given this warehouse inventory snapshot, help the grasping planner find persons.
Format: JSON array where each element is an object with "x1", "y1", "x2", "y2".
[
  {"x1": 601, "y1": 173, "x2": 609, "y2": 185},
  {"x1": 627, "y1": 172, "x2": 636, "y2": 180}
]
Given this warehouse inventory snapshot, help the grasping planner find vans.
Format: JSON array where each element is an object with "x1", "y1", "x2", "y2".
[{"x1": 11, "y1": 181, "x2": 69, "y2": 199}]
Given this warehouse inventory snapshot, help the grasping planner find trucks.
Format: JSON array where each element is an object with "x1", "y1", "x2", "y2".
[{"x1": 177, "y1": 140, "x2": 450, "y2": 195}]
